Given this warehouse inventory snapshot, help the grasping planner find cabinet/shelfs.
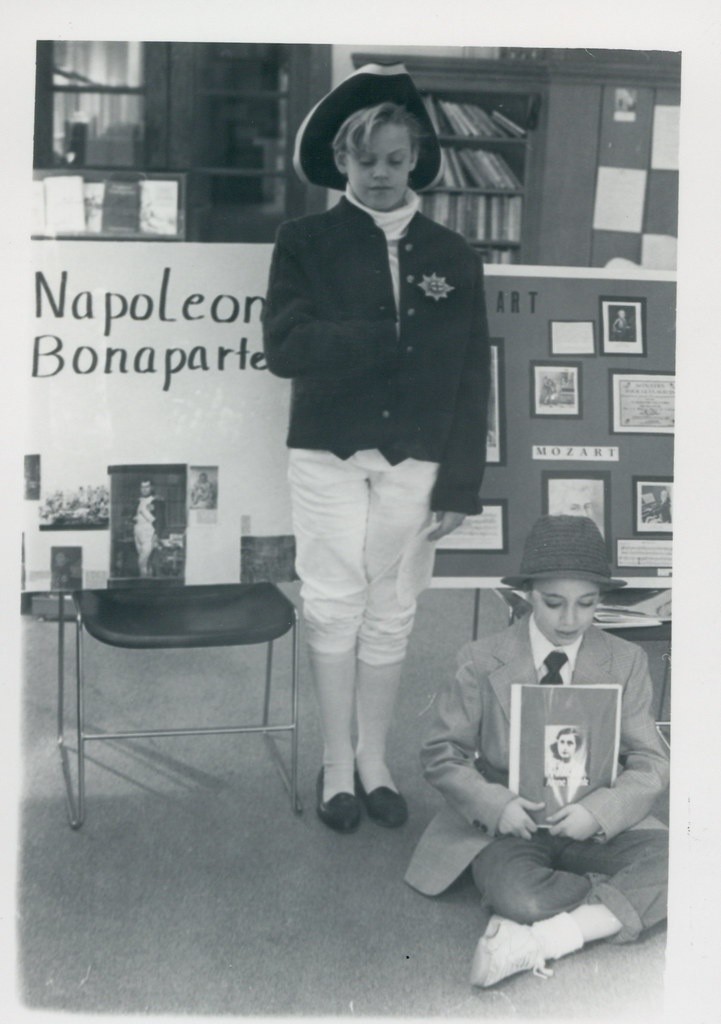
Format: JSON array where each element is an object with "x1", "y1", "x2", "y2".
[{"x1": 350, "y1": 52, "x2": 553, "y2": 269}]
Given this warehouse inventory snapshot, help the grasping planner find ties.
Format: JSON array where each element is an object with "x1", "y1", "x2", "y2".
[{"x1": 539, "y1": 652, "x2": 568, "y2": 686}]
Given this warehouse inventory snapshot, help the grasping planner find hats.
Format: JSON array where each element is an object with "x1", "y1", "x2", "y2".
[
  {"x1": 501, "y1": 513, "x2": 628, "y2": 589},
  {"x1": 293, "y1": 60, "x2": 447, "y2": 193}
]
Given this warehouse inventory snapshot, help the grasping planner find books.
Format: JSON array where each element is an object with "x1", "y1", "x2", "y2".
[
  {"x1": 507, "y1": 682, "x2": 621, "y2": 830},
  {"x1": 419, "y1": 94, "x2": 527, "y2": 266}
]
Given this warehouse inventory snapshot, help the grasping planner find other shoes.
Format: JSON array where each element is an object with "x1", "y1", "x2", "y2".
[
  {"x1": 470, "y1": 914, "x2": 553, "y2": 990},
  {"x1": 316, "y1": 764, "x2": 360, "y2": 833},
  {"x1": 358, "y1": 769, "x2": 407, "y2": 827}
]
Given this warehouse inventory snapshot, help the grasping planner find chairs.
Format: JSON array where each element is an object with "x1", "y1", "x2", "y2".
[
  {"x1": 472, "y1": 585, "x2": 671, "y2": 727},
  {"x1": 57, "y1": 584, "x2": 304, "y2": 830}
]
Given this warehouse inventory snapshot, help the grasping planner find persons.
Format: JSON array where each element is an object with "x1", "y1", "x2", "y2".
[
  {"x1": 399, "y1": 516, "x2": 667, "y2": 990},
  {"x1": 544, "y1": 728, "x2": 590, "y2": 785},
  {"x1": 644, "y1": 490, "x2": 671, "y2": 523},
  {"x1": 191, "y1": 470, "x2": 214, "y2": 507},
  {"x1": 260, "y1": 64, "x2": 493, "y2": 834},
  {"x1": 610, "y1": 310, "x2": 633, "y2": 339},
  {"x1": 126, "y1": 478, "x2": 170, "y2": 578}
]
